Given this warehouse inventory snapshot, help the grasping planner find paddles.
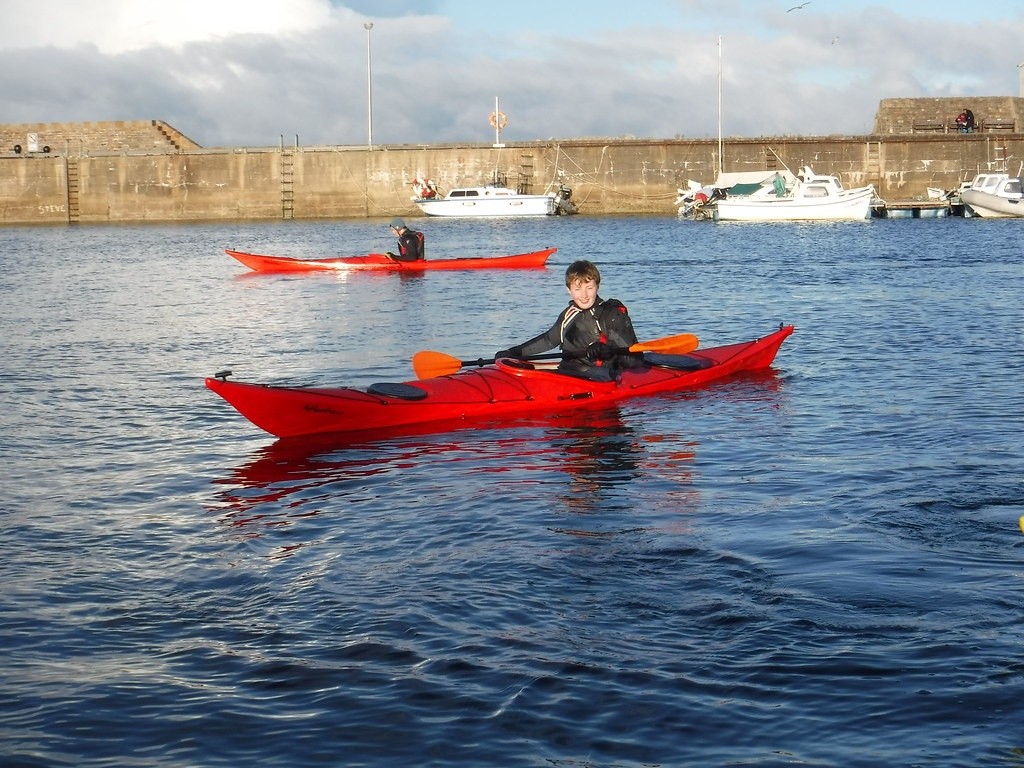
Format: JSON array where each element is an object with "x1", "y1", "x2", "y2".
[
  {"x1": 412, "y1": 332, "x2": 699, "y2": 380},
  {"x1": 383, "y1": 251, "x2": 402, "y2": 267}
]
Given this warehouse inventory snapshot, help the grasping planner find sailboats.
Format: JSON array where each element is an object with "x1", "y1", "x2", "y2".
[
  {"x1": 695, "y1": 32, "x2": 878, "y2": 222},
  {"x1": 953, "y1": 135, "x2": 1024, "y2": 219}
]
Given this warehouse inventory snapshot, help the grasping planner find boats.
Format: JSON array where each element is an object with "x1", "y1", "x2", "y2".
[
  {"x1": 413, "y1": 178, "x2": 580, "y2": 219},
  {"x1": 224, "y1": 244, "x2": 557, "y2": 274},
  {"x1": 204, "y1": 322, "x2": 795, "y2": 449}
]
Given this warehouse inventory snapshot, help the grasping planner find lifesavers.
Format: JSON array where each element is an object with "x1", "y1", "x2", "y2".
[
  {"x1": 488, "y1": 111, "x2": 508, "y2": 129},
  {"x1": 412, "y1": 176, "x2": 437, "y2": 197}
]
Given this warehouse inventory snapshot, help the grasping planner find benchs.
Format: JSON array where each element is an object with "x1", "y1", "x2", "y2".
[{"x1": 913, "y1": 118, "x2": 1017, "y2": 133}]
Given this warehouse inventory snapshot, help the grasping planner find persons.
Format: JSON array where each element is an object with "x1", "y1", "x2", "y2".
[
  {"x1": 495, "y1": 261, "x2": 644, "y2": 383},
  {"x1": 956, "y1": 110, "x2": 974, "y2": 133},
  {"x1": 385, "y1": 217, "x2": 425, "y2": 262}
]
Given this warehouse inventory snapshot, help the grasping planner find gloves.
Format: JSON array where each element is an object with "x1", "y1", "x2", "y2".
[
  {"x1": 586, "y1": 342, "x2": 615, "y2": 361},
  {"x1": 495, "y1": 350, "x2": 511, "y2": 361}
]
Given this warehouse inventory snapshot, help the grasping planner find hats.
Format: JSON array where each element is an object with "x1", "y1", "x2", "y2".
[{"x1": 390, "y1": 217, "x2": 405, "y2": 232}]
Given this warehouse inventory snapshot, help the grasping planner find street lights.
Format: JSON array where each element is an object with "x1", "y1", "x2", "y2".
[{"x1": 364, "y1": 21, "x2": 374, "y2": 140}]
